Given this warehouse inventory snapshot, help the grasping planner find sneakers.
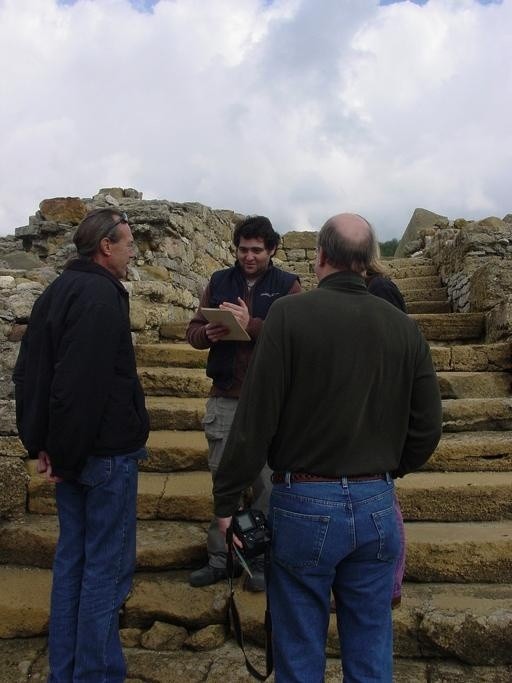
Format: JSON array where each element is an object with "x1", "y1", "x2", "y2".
[
  {"x1": 190, "y1": 565, "x2": 240, "y2": 587},
  {"x1": 246, "y1": 570, "x2": 265, "y2": 592}
]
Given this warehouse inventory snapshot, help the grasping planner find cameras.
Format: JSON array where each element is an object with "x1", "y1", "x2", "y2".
[{"x1": 232, "y1": 509, "x2": 272, "y2": 558}]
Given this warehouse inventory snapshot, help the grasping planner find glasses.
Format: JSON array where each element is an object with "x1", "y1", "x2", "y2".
[{"x1": 104, "y1": 212, "x2": 128, "y2": 236}]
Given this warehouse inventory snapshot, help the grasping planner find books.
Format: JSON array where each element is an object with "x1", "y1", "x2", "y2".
[{"x1": 200, "y1": 305, "x2": 253, "y2": 344}]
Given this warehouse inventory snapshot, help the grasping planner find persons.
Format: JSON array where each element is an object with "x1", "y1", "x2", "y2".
[
  {"x1": 210, "y1": 211, "x2": 445, "y2": 682},
  {"x1": 328, "y1": 225, "x2": 407, "y2": 609},
  {"x1": 183, "y1": 213, "x2": 299, "y2": 592},
  {"x1": 11, "y1": 205, "x2": 150, "y2": 682}
]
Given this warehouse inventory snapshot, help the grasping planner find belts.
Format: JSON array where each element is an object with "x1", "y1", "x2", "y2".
[{"x1": 272, "y1": 472, "x2": 386, "y2": 484}]
